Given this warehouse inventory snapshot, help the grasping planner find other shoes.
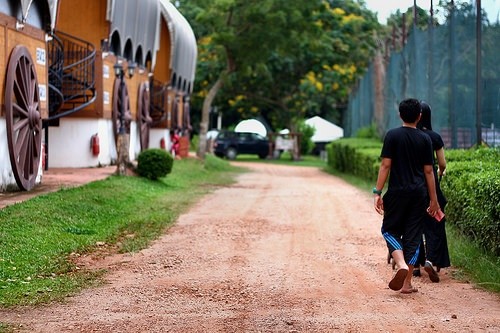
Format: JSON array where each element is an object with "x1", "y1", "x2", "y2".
[
  {"x1": 424, "y1": 261, "x2": 440, "y2": 282},
  {"x1": 412, "y1": 269, "x2": 421, "y2": 277}
]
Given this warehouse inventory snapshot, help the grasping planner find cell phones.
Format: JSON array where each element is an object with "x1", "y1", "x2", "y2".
[{"x1": 427, "y1": 206, "x2": 445, "y2": 222}]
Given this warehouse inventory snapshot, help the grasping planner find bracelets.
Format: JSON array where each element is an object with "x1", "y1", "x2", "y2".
[{"x1": 373, "y1": 187, "x2": 382, "y2": 195}]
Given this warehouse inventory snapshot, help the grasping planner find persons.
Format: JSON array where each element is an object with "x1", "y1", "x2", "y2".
[
  {"x1": 374, "y1": 98, "x2": 442, "y2": 294},
  {"x1": 412, "y1": 101, "x2": 451, "y2": 284}
]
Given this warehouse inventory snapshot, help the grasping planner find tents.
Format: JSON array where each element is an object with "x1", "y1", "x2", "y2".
[{"x1": 304, "y1": 115, "x2": 344, "y2": 143}]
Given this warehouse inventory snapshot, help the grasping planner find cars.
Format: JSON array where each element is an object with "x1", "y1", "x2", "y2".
[{"x1": 212, "y1": 131, "x2": 269, "y2": 159}]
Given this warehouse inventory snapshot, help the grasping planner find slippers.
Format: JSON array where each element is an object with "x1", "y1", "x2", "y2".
[{"x1": 387, "y1": 267, "x2": 419, "y2": 293}]
